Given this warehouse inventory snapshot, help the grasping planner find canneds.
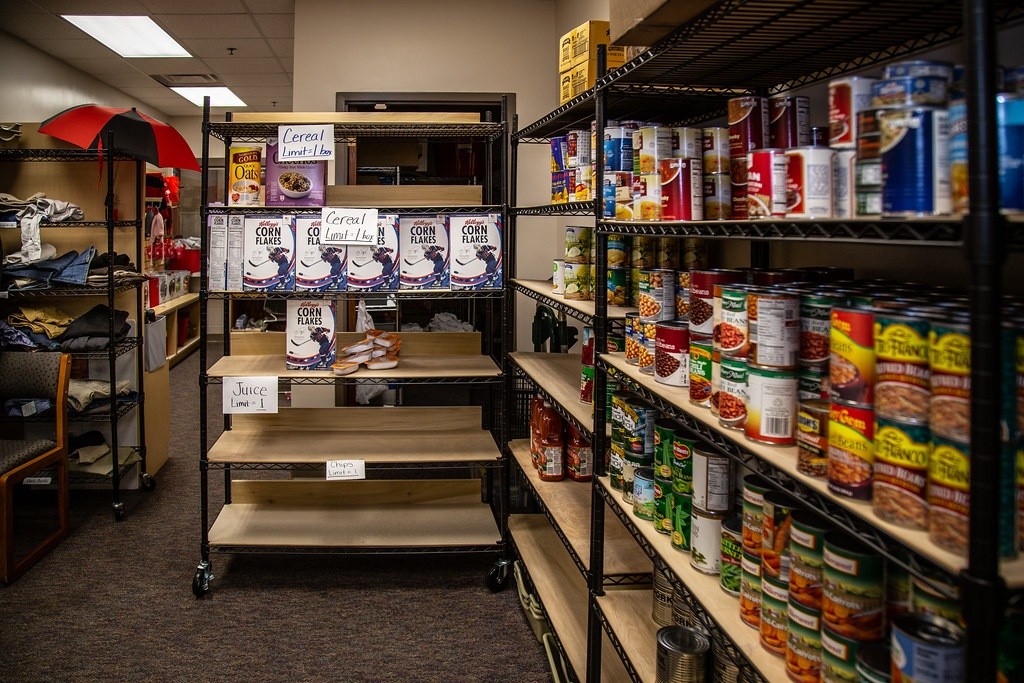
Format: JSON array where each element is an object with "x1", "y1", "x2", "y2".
[{"x1": 550, "y1": 60, "x2": 1024, "y2": 683}]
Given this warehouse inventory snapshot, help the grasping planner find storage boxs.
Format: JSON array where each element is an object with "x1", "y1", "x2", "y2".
[{"x1": 558, "y1": 0, "x2": 719, "y2": 105}]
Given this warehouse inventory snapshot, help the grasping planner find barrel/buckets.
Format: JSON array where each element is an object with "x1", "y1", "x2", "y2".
[{"x1": 170, "y1": 248, "x2": 201, "y2": 273}]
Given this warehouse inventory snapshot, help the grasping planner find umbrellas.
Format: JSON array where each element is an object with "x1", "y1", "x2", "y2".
[{"x1": 36, "y1": 102, "x2": 204, "y2": 222}]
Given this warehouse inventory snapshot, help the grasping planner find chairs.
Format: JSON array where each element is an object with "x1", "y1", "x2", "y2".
[{"x1": 0, "y1": 352, "x2": 72, "y2": 589}]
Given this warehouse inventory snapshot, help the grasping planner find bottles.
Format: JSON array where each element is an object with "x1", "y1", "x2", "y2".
[{"x1": 529, "y1": 394, "x2": 593, "y2": 483}]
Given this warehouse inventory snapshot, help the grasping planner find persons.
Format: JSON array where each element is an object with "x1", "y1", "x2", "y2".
[{"x1": 145, "y1": 203, "x2": 165, "y2": 271}]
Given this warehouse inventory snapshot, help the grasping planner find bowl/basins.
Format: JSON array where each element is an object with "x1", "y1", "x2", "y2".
[
  {"x1": 278, "y1": 171, "x2": 313, "y2": 198},
  {"x1": 639, "y1": 295, "x2": 970, "y2": 683},
  {"x1": 232, "y1": 180, "x2": 261, "y2": 204}
]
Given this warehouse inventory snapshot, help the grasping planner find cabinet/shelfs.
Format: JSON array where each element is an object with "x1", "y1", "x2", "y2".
[
  {"x1": 142, "y1": 171, "x2": 202, "y2": 369},
  {"x1": 0, "y1": 130, "x2": 154, "y2": 523},
  {"x1": 508, "y1": 0, "x2": 1024, "y2": 683},
  {"x1": 193, "y1": 97, "x2": 517, "y2": 598}
]
